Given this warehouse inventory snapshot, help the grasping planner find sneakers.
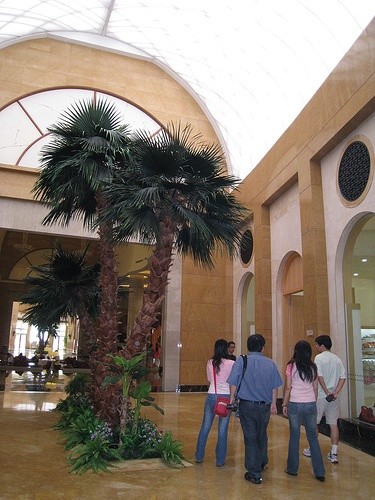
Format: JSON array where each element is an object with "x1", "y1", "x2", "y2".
[
  {"x1": 326, "y1": 450, "x2": 339, "y2": 463},
  {"x1": 303, "y1": 448, "x2": 312, "y2": 457}
]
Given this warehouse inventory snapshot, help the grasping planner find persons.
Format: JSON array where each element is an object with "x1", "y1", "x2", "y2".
[
  {"x1": 225, "y1": 341, "x2": 236, "y2": 361},
  {"x1": 303, "y1": 335, "x2": 346, "y2": 463},
  {"x1": 282, "y1": 341, "x2": 326, "y2": 482},
  {"x1": 226, "y1": 334, "x2": 283, "y2": 484},
  {"x1": 195, "y1": 338, "x2": 235, "y2": 467}
]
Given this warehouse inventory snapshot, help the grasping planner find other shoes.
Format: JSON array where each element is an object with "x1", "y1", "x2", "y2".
[
  {"x1": 262, "y1": 463, "x2": 265, "y2": 471},
  {"x1": 216, "y1": 463, "x2": 224, "y2": 467},
  {"x1": 196, "y1": 460, "x2": 203, "y2": 463},
  {"x1": 245, "y1": 472, "x2": 262, "y2": 484},
  {"x1": 284, "y1": 468, "x2": 297, "y2": 476},
  {"x1": 316, "y1": 475, "x2": 325, "y2": 482}
]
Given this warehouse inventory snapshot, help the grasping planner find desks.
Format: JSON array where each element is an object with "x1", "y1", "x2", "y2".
[
  {"x1": 0, "y1": 365, "x2": 43, "y2": 391},
  {"x1": 63, "y1": 368, "x2": 90, "y2": 374}
]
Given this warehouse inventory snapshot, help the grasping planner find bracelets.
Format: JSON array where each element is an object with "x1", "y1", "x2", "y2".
[{"x1": 282, "y1": 404, "x2": 288, "y2": 407}]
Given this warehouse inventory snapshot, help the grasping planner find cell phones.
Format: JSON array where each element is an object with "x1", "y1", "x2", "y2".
[{"x1": 326, "y1": 394, "x2": 334, "y2": 402}]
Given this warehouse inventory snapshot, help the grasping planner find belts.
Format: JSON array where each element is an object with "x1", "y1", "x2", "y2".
[{"x1": 240, "y1": 398, "x2": 265, "y2": 404}]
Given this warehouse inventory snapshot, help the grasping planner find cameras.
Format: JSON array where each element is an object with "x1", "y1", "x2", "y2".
[{"x1": 226, "y1": 401, "x2": 239, "y2": 412}]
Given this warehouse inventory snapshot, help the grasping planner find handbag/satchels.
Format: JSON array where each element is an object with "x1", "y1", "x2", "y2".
[{"x1": 214, "y1": 397, "x2": 230, "y2": 417}]
